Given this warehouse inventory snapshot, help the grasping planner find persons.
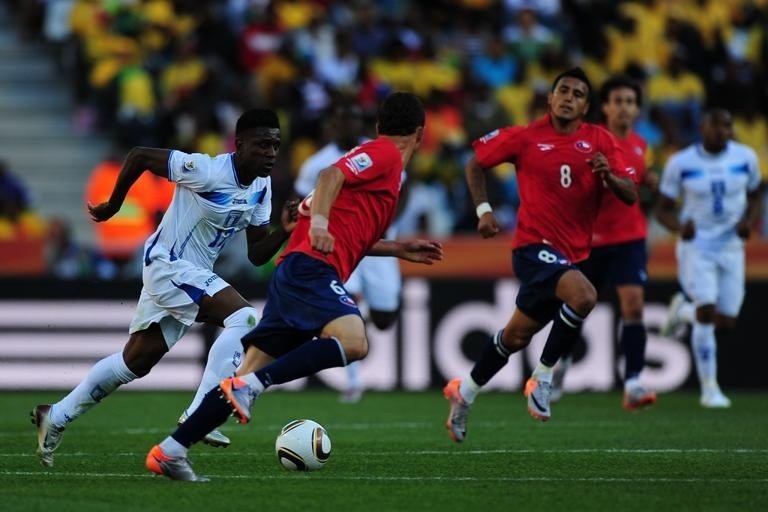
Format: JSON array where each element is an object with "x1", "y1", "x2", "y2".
[
  {"x1": 442, "y1": 67, "x2": 659, "y2": 443},
  {"x1": 0, "y1": 0, "x2": 767, "y2": 282},
  {"x1": 31, "y1": 93, "x2": 444, "y2": 482},
  {"x1": 654, "y1": 107, "x2": 762, "y2": 409}
]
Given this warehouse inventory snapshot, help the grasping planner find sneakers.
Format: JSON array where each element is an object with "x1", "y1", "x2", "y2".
[
  {"x1": 177, "y1": 410, "x2": 231, "y2": 448},
  {"x1": 144, "y1": 444, "x2": 210, "y2": 483},
  {"x1": 443, "y1": 378, "x2": 469, "y2": 442},
  {"x1": 623, "y1": 386, "x2": 656, "y2": 411},
  {"x1": 30, "y1": 404, "x2": 65, "y2": 468},
  {"x1": 699, "y1": 385, "x2": 731, "y2": 408},
  {"x1": 523, "y1": 376, "x2": 551, "y2": 421},
  {"x1": 660, "y1": 292, "x2": 686, "y2": 338},
  {"x1": 217, "y1": 376, "x2": 257, "y2": 425}
]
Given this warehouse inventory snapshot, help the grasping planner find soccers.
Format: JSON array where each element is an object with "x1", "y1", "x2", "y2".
[{"x1": 276, "y1": 418, "x2": 332, "y2": 472}]
